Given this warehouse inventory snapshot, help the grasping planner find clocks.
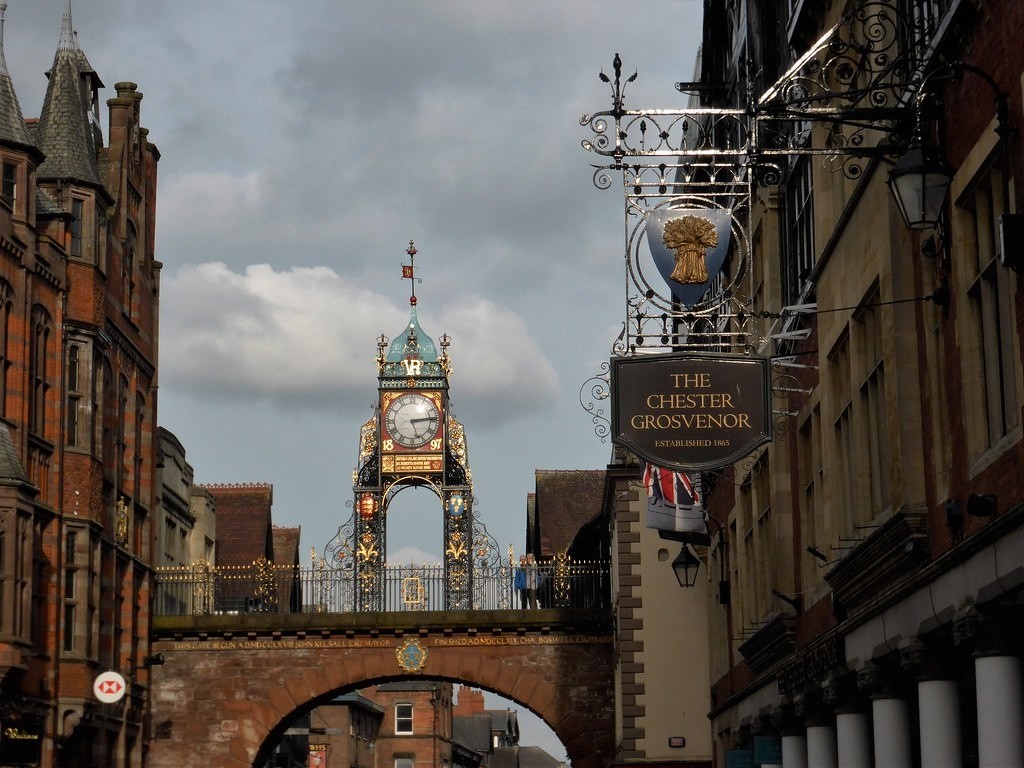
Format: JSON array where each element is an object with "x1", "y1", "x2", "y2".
[{"x1": 385, "y1": 394, "x2": 440, "y2": 448}]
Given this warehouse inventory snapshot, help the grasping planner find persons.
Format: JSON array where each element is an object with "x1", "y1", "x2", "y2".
[
  {"x1": 526, "y1": 553, "x2": 541, "y2": 610},
  {"x1": 514, "y1": 555, "x2": 527, "y2": 609}
]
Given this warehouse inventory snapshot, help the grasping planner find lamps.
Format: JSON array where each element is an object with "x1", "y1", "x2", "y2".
[
  {"x1": 885, "y1": 61, "x2": 1024, "y2": 269},
  {"x1": 143, "y1": 651, "x2": 165, "y2": 668},
  {"x1": 672, "y1": 514, "x2": 732, "y2": 604},
  {"x1": 965, "y1": 491, "x2": 995, "y2": 517}
]
